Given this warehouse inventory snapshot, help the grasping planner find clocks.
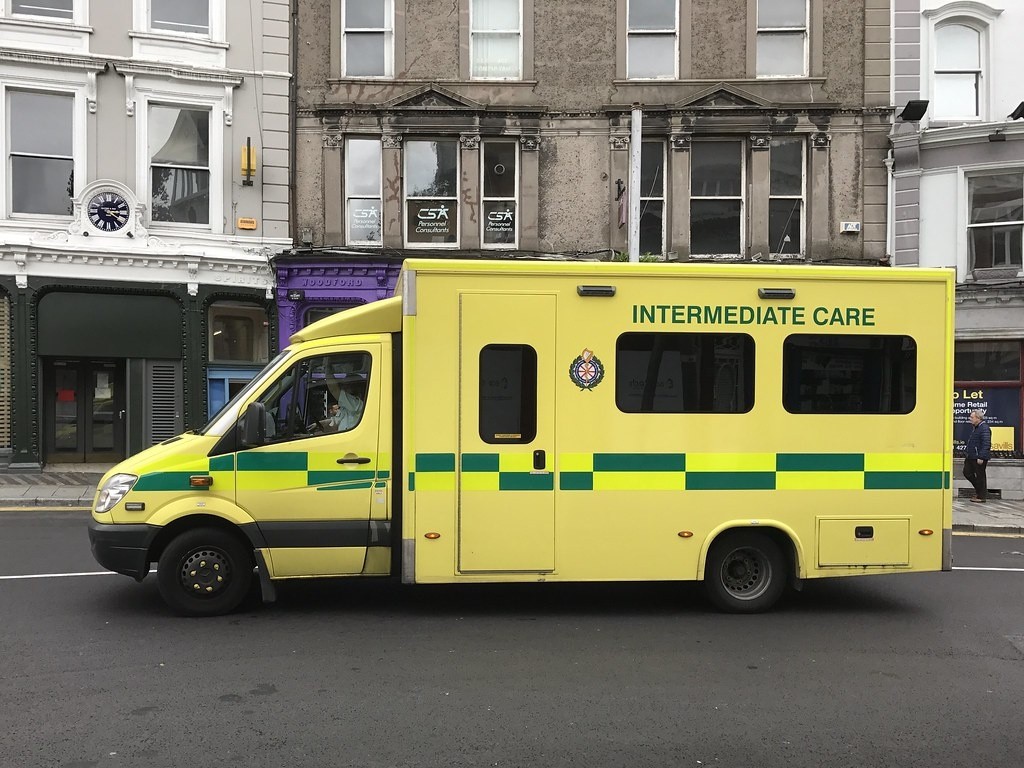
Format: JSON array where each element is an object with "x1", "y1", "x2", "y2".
[{"x1": 88, "y1": 191, "x2": 132, "y2": 233}]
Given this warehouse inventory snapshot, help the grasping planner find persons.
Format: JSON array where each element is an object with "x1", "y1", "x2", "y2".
[
  {"x1": 961, "y1": 409, "x2": 993, "y2": 503},
  {"x1": 304, "y1": 357, "x2": 366, "y2": 433}
]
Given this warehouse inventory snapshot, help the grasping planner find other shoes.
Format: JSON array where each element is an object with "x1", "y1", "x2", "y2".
[{"x1": 970, "y1": 494, "x2": 986, "y2": 503}]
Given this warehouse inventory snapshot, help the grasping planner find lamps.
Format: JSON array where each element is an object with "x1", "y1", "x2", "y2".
[
  {"x1": 1006, "y1": 102, "x2": 1024, "y2": 121},
  {"x1": 896, "y1": 100, "x2": 929, "y2": 121},
  {"x1": 668, "y1": 251, "x2": 678, "y2": 263},
  {"x1": 751, "y1": 252, "x2": 762, "y2": 263}
]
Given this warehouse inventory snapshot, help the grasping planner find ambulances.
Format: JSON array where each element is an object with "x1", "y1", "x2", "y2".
[{"x1": 86, "y1": 261, "x2": 956, "y2": 616}]
